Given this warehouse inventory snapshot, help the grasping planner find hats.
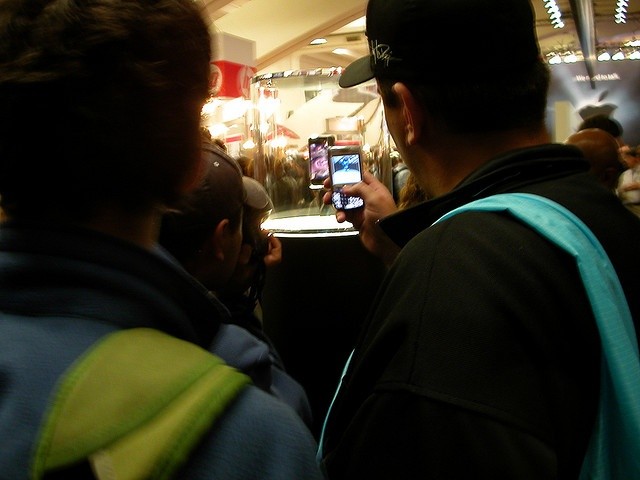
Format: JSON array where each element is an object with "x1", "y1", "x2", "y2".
[
  {"x1": 243, "y1": 176, "x2": 274, "y2": 214},
  {"x1": 338, "y1": 0, "x2": 540, "y2": 88},
  {"x1": 167, "y1": 137, "x2": 247, "y2": 234}
]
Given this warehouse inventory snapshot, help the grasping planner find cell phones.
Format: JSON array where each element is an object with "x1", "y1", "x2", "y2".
[
  {"x1": 328, "y1": 152, "x2": 365, "y2": 211},
  {"x1": 308, "y1": 134, "x2": 335, "y2": 190}
]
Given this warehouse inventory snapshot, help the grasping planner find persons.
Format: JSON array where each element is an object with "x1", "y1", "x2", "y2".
[
  {"x1": 241, "y1": 176, "x2": 282, "y2": 301},
  {"x1": 0, "y1": 1, "x2": 320, "y2": 480},
  {"x1": 617, "y1": 144, "x2": 639, "y2": 218},
  {"x1": 155, "y1": 141, "x2": 242, "y2": 286},
  {"x1": 577, "y1": 115, "x2": 624, "y2": 149},
  {"x1": 563, "y1": 128, "x2": 618, "y2": 196},
  {"x1": 317, "y1": 0, "x2": 640, "y2": 479}
]
